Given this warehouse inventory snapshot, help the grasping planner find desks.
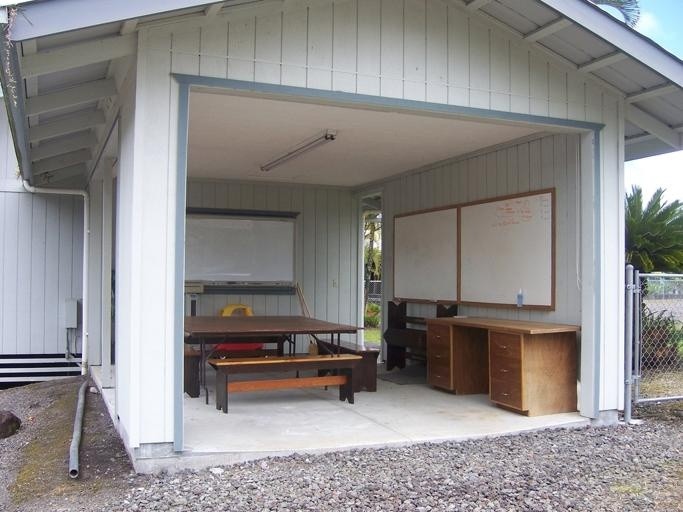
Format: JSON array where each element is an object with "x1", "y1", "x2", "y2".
[
  {"x1": 426, "y1": 317, "x2": 581, "y2": 417},
  {"x1": 184, "y1": 316, "x2": 365, "y2": 404}
]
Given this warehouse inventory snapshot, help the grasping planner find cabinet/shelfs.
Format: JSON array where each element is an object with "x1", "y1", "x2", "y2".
[{"x1": 382, "y1": 301, "x2": 457, "y2": 371}]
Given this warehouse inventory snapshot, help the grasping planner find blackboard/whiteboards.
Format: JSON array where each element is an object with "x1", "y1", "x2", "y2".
[
  {"x1": 392, "y1": 188, "x2": 557, "y2": 312},
  {"x1": 185, "y1": 208, "x2": 300, "y2": 295}
]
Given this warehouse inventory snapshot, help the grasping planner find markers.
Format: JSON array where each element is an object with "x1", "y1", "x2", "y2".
[
  {"x1": 272, "y1": 283, "x2": 279, "y2": 285},
  {"x1": 227, "y1": 281, "x2": 244, "y2": 284}
]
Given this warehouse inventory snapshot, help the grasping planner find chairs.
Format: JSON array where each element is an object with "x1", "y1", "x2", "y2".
[{"x1": 215, "y1": 304, "x2": 263, "y2": 356}]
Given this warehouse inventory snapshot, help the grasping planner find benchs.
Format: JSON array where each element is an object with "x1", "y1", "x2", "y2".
[
  {"x1": 208, "y1": 353, "x2": 363, "y2": 413},
  {"x1": 184, "y1": 344, "x2": 201, "y2": 398},
  {"x1": 184, "y1": 336, "x2": 287, "y2": 356},
  {"x1": 317, "y1": 339, "x2": 381, "y2": 393}
]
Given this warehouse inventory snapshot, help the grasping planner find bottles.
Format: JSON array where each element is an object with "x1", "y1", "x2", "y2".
[{"x1": 515, "y1": 289, "x2": 522, "y2": 306}]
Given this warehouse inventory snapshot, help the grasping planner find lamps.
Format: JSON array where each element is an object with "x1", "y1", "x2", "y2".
[{"x1": 260, "y1": 129, "x2": 336, "y2": 172}]
